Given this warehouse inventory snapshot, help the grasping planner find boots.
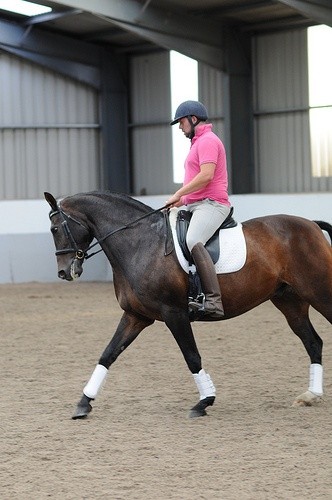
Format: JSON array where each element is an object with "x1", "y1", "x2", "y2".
[{"x1": 188, "y1": 242, "x2": 224, "y2": 318}]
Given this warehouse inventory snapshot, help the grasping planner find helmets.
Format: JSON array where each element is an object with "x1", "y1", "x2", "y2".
[{"x1": 171, "y1": 100, "x2": 208, "y2": 125}]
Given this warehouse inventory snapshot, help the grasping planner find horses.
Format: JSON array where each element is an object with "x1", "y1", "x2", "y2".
[{"x1": 43, "y1": 190, "x2": 332, "y2": 421}]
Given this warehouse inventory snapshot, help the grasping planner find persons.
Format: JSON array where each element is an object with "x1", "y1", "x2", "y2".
[{"x1": 163, "y1": 100, "x2": 232, "y2": 319}]
